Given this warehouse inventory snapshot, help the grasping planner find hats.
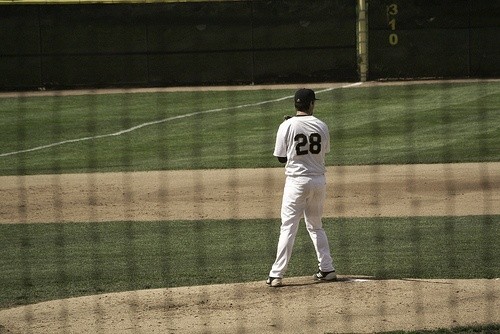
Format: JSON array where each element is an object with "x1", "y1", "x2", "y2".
[{"x1": 294, "y1": 88, "x2": 320, "y2": 102}]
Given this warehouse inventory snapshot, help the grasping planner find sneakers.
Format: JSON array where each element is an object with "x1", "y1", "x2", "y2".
[
  {"x1": 265, "y1": 277, "x2": 283, "y2": 287},
  {"x1": 312, "y1": 271, "x2": 337, "y2": 281}
]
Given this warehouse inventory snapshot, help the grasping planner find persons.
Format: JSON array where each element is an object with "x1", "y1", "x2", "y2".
[{"x1": 266, "y1": 88, "x2": 337, "y2": 287}]
